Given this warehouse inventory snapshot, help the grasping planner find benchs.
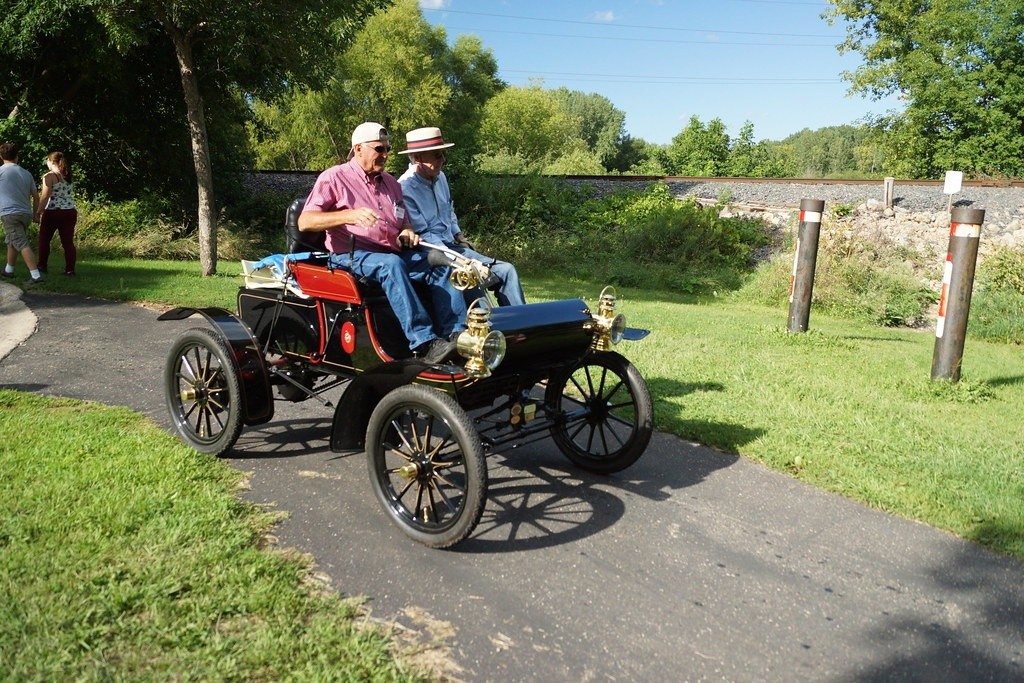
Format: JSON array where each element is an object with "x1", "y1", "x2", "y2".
[{"x1": 286, "y1": 199, "x2": 380, "y2": 304}]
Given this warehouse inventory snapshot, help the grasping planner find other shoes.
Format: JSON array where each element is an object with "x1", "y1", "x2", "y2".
[
  {"x1": 0, "y1": 268, "x2": 14, "y2": 278},
  {"x1": 35, "y1": 265, "x2": 47, "y2": 273},
  {"x1": 63, "y1": 271, "x2": 75, "y2": 276},
  {"x1": 20, "y1": 277, "x2": 45, "y2": 287}
]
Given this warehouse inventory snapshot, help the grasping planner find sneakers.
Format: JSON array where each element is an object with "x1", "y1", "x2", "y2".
[
  {"x1": 414, "y1": 336, "x2": 458, "y2": 366},
  {"x1": 449, "y1": 331, "x2": 462, "y2": 343}
]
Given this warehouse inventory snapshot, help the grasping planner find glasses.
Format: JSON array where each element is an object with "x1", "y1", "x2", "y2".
[
  {"x1": 423, "y1": 150, "x2": 448, "y2": 159},
  {"x1": 360, "y1": 143, "x2": 392, "y2": 154}
]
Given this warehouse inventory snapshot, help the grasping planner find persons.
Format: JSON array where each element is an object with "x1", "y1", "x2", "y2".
[
  {"x1": 37, "y1": 152, "x2": 77, "y2": 275},
  {"x1": 397, "y1": 127, "x2": 526, "y2": 308},
  {"x1": 298, "y1": 122, "x2": 467, "y2": 363},
  {"x1": 0, "y1": 143, "x2": 44, "y2": 284}
]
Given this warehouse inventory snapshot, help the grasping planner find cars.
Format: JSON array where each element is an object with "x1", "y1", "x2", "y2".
[{"x1": 154, "y1": 196, "x2": 655, "y2": 552}]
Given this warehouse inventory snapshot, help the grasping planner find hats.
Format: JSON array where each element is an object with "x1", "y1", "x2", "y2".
[
  {"x1": 346, "y1": 121, "x2": 390, "y2": 160},
  {"x1": 398, "y1": 127, "x2": 454, "y2": 154}
]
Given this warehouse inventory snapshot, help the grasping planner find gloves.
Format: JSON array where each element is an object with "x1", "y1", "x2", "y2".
[
  {"x1": 459, "y1": 238, "x2": 475, "y2": 249},
  {"x1": 469, "y1": 260, "x2": 501, "y2": 290}
]
